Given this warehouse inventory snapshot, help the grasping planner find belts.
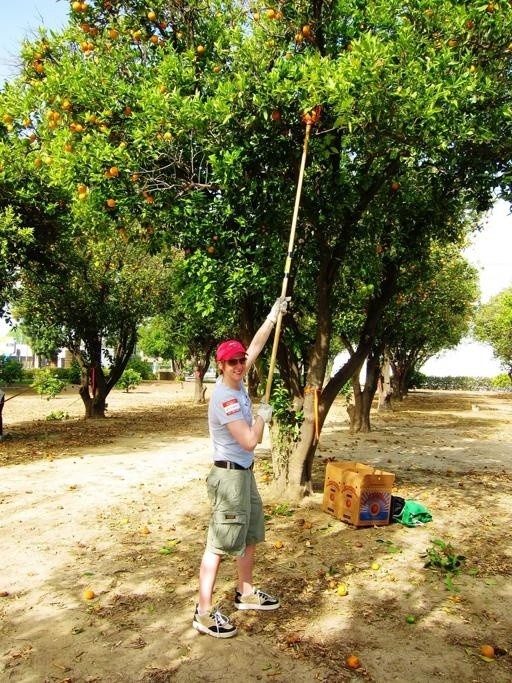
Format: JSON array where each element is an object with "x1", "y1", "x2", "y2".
[{"x1": 214, "y1": 460, "x2": 255, "y2": 471}]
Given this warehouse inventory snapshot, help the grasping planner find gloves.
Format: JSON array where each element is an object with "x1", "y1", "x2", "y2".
[
  {"x1": 267, "y1": 296, "x2": 292, "y2": 324},
  {"x1": 255, "y1": 395, "x2": 273, "y2": 424}
]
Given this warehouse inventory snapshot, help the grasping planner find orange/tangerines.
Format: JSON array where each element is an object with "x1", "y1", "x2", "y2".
[
  {"x1": 143, "y1": 527, "x2": 149, "y2": 534},
  {"x1": 407, "y1": 616, "x2": 414, "y2": 624},
  {"x1": 372, "y1": 563, "x2": 379, "y2": 570},
  {"x1": 338, "y1": 585, "x2": 346, "y2": 595},
  {"x1": 275, "y1": 541, "x2": 283, "y2": 548},
  {"x1": 346, "y1": 655, "x2": 360, "y2": 668},
  {"x1": 84, "y1": 591, "x2": 94, "y2": 599},
  {"x1": 482, "y1": 646, "x2": 494, "y2": 657},
  {"x1": 4, "y1": 0, "x2": 512, "y2": 256}
]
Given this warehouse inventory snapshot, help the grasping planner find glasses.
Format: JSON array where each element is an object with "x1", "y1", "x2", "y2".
[{"x1": 225, "y1": 356, "x2": 248, "y2": 366}]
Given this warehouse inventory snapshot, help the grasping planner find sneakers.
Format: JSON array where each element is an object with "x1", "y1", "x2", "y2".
[
  {"x1": 192, "y1": 603, "x2": 237, "y2": 639},
  {"x1": 234, "y1": 586, "x2": 281, "y2": 610}
]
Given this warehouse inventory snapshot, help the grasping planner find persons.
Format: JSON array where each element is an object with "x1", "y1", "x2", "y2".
[
  {"x1": 0, "y1": 388, "x2": 5, "y2": 443},
  {"x1": 192, "y1": 295, "x2": 293, "y2": 638}
]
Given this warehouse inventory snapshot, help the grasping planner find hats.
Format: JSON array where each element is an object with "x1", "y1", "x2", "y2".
[{"x1": 216, "y1": 340, "x2": 248, "y2": 361}]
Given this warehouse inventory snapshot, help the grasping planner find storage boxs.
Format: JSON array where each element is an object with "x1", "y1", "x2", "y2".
[{"x1": 323, "y1": 461, "x2": 395, "y2": 528}]
